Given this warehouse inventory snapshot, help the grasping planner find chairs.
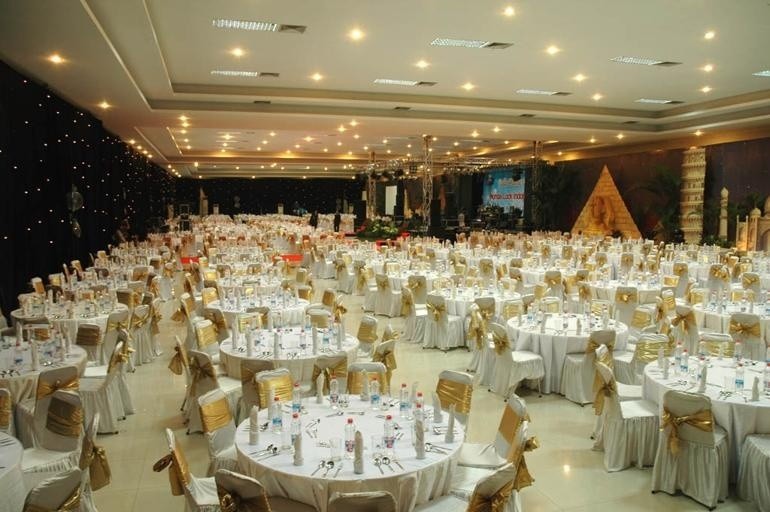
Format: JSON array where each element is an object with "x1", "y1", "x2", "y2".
[{"x1": 0, "y1": 212, "x2": 770, "y2": 512}]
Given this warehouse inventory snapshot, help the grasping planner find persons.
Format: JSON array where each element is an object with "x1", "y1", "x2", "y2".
[
  {"x1": 590, "y1": 195, "x2": 616, "y2": 235},
  {"x1": 310, "y1": 210, "x2": 318, "y2": 229},
  {"x1": 334, "y1": 210, "x2": 341, "y2": 232}
]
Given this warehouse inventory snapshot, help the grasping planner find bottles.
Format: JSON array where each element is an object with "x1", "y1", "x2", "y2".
[
  {"x1": 0, "y1": 274, "x2": 119, "y2": 384},
  {"x1": 248, "y1": 373, "x2": 460, "y2": 482},
  {"x1": 217, "y1": 274, "x2": 346, "y2": 361},
  {"x1": 654, "y1": 339, "x2": 769, "y2": 404},
  {"x1": 514, "y1": 295, "x2": 626, "y2": 343}
]
